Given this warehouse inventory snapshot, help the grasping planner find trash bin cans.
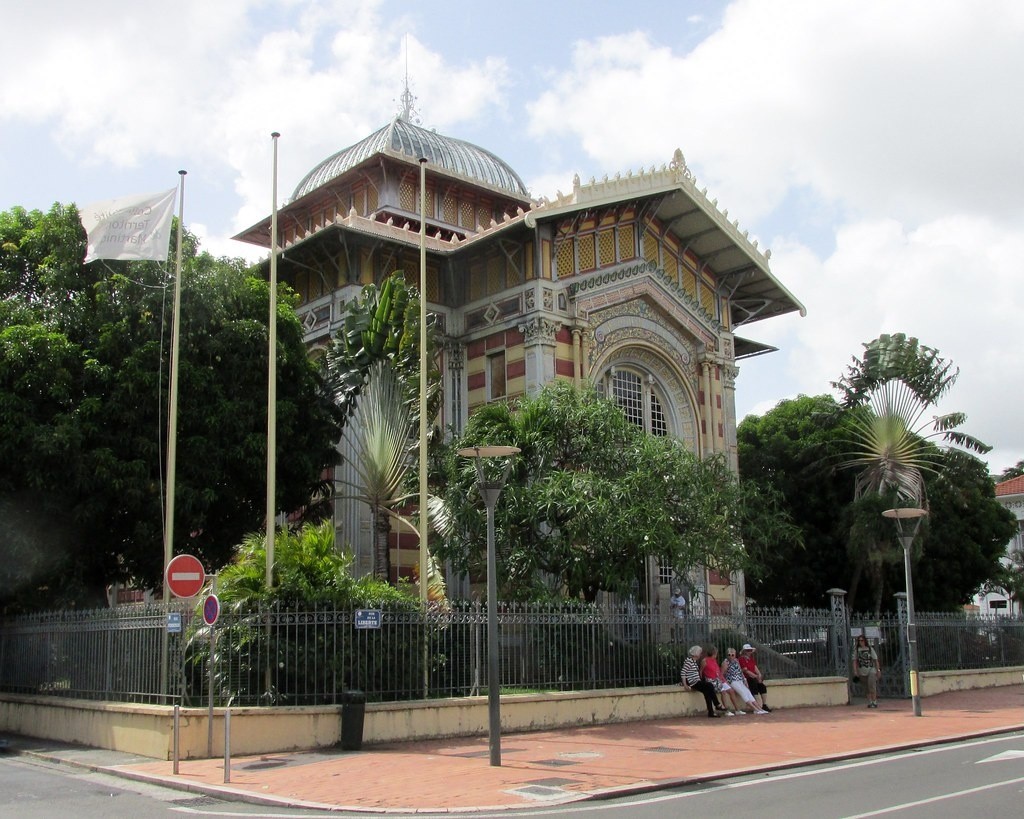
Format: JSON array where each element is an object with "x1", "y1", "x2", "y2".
[{"x1": 342, "y1": 691, "x2": 366, "y2": 751}]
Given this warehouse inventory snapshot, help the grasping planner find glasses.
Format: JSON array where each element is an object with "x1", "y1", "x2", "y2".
[
  {"x1": 727, "y1": 654, "x2": 735, "y2": 657},
  {"x1": 859, "y1": 639, "x2": 864, "y2": 642},
  {"x1": 744, "y1": 650, "x2": 752, "y2": 652}
]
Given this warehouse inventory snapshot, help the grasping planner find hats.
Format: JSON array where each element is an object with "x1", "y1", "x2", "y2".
[{"x1": 739, "y1": 644, "x2": 756, "y2": 654}]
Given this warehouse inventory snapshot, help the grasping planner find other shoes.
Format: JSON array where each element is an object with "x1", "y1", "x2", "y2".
[
  {"x1": 873, "y1": 699, "x2": 878, "y2": 707},
  {"x1": 754, "y1": 710, "x2": 764, "y2": 714},
  {"x1": 716, "y1": 705, "x2": 728, "y2": 710},
  {"x1": 709, "y1": 714, "x2": 720, "y2": 718},
  {"x1": 725, "y1": 712, "x2": 734, "y2": 716},
  {"x1": 763, "y1": 706, "x2": 771, "y2": 712},
  {"x1": 761, "y1": 710, "x2": 769, "y2": 713},
  {"x1": 868, "y1": 699, "x2": 872, "y2": 708},
  {"x1": 735, "y1": 710, "x2": 747, "y2": 715}
]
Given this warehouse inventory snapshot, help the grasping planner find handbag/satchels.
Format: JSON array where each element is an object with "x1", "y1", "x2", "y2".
[{"x1": 749, "y1": 679, "x2": 766, "y2": 695}]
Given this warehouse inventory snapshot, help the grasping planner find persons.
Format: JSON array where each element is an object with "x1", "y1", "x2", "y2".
[
  {"x1": 739, "y1": 644, "x2": 773, "y2": 712},
  {"x1": 699, "y1": 645, "x2": 746, "y2": 717},
  {"x1": 680, "y1": 646, "x2": 728, "y2": 718},
  {"x1": 721, "y1": 647, "x2": 769, "y2": 715},
  {"x1": 853, "y1": 635, "x2": 883, "y2": 706}
]
